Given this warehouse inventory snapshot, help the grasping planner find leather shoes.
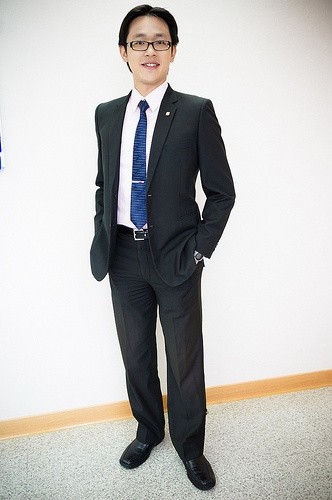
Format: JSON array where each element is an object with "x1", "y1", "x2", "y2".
[
  {"x1": 183, "y1": 455, "x2": 216, "y2": 491},
  {"x1": 118, "y1": 438, "x2": 158, "y2": 469}
]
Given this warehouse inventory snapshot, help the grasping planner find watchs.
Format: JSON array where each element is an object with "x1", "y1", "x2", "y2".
[{"x1": 193, "y1": 250, "x2": 204, "y2": 262}]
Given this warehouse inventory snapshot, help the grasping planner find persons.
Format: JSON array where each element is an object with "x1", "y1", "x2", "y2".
[{"x1": 89, "y1": 5, "x2": 237, "y2": 491}]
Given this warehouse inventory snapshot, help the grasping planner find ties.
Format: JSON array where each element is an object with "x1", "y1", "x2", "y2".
[{"x1": 129, "y1": 99, "x2": 150, "y2": 231}]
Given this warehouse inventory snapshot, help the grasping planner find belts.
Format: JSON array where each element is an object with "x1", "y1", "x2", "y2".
[{"x1": 118, "y1": 226, "x2": 147, "y2": 240}]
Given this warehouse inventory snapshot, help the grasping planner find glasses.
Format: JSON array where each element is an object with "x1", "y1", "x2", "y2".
[{"x1": 125, "y1": 40, "x2": 173, "y2": 51}]
